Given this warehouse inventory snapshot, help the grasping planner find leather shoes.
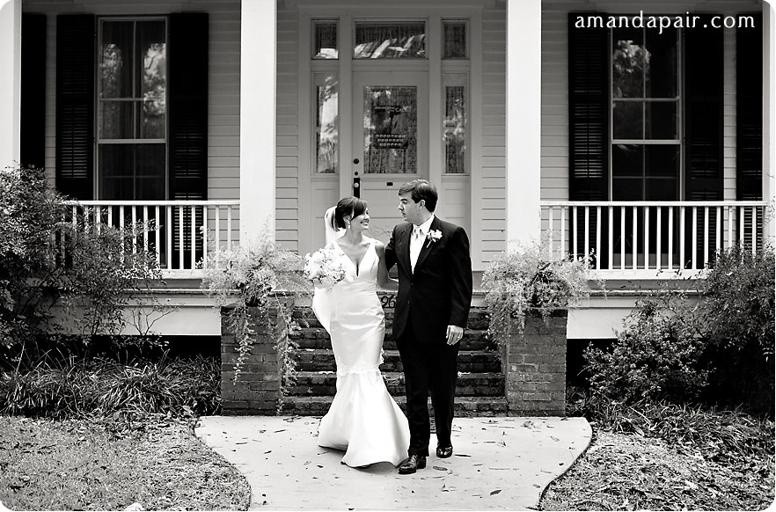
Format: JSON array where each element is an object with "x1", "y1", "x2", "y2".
[
  {"x1": 399, "y1": 454, "x2": 426, "y2": 473},
  {"x1": 436, "y1": 442, "x2": 452, "y2": 457}
]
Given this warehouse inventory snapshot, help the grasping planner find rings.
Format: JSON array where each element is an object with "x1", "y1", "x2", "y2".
[{"x1": 457, "y1": 336, "x2": 460, "y2": 339}]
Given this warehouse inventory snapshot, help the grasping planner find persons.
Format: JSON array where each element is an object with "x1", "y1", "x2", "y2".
[
  {"x1": 307, "y1": 195, "x2": 401, "y2": 470},
  {"x1": 384, "y1": 178, "x2": 472, "y2": 474}
]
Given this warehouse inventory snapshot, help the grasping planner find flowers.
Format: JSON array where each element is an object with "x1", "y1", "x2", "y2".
[
  {"x1": 426, "y1": 228, "x2": 442, "y2": 248},
  {"x1": 303, "y1": 247, "x2": 347, "y2": 292}
]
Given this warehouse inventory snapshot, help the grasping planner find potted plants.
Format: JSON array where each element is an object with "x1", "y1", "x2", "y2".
[
  {"x1": 193, "y1": 221, "x2": 306, "y2": 416},
  {"x1": 481, "y1": 235, "x2": 610, "y2": 418}
]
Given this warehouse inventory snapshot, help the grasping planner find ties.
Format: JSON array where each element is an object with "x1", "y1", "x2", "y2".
[{"x1": 409, "y1": 227, "x2": 422, "y2": 272}]
